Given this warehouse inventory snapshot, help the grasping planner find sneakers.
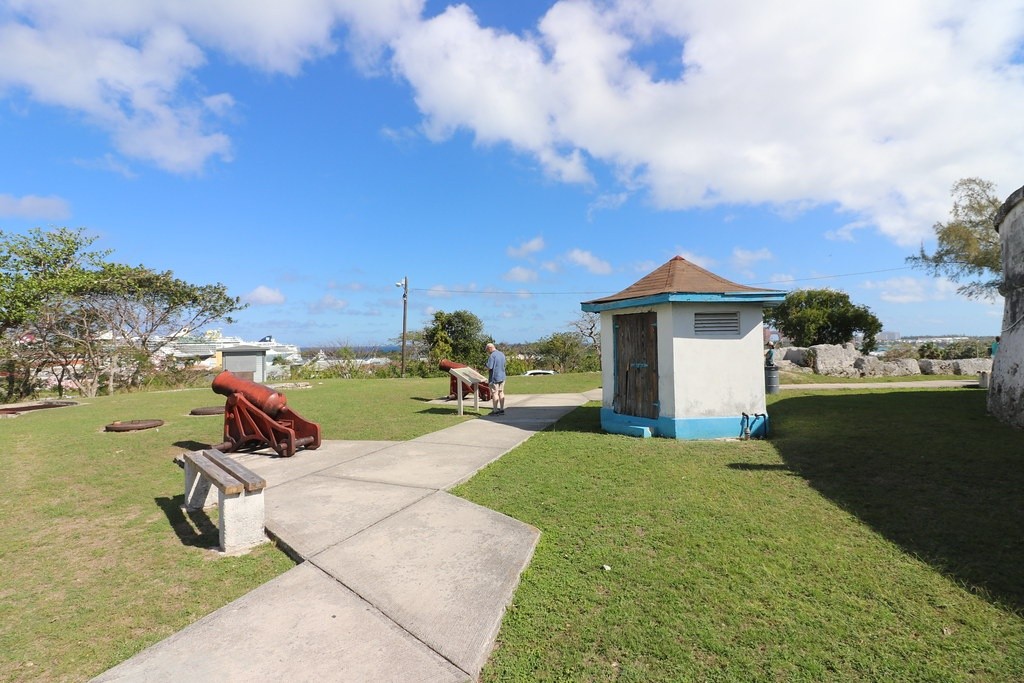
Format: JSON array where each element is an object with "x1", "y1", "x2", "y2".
[{"x1": 488, "y1": 410, "x2": 504, "y2": 416}]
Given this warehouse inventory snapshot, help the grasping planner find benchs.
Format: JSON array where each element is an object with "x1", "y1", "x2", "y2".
[{"x1": 183, "y1": 448, "x2": 266, "y2": 554}]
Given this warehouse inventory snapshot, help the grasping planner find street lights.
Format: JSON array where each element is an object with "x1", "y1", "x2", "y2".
[{"x1": 397, "y1": 277, "x2": 408, "y2": 376}]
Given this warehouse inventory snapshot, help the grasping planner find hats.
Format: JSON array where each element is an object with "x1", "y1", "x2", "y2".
[
  {"x1": 766, "y1": 341, "x2": 773, "y2": 346},
  {"x1": 484, "y1": 343, "x2": 495, "y2": 351}
]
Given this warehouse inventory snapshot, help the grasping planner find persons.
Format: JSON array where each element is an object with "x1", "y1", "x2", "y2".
[
  {"x1": 485, "y1": 343, "x2": 506, "y2": 417},
  {"x1": 990, "y1": 336, "x2": 1001, "y2": 362},
  {"x1": 764, "y1": 340, "x2": 775, "y2": 366}
]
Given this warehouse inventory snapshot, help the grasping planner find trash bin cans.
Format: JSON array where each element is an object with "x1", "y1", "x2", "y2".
[{"x1": 977, "y1": 370, "x2": 992, "y2": 387}]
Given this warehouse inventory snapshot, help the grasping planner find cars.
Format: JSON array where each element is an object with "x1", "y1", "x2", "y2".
[{"x1": 521, "y1": 369, "x2": 557, "y2": 376}]
